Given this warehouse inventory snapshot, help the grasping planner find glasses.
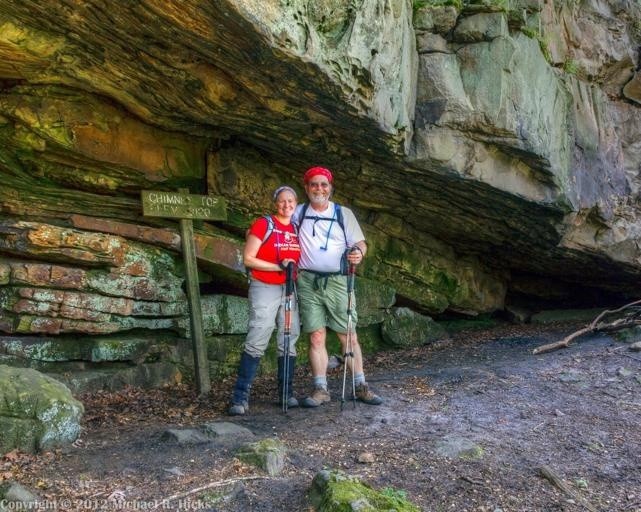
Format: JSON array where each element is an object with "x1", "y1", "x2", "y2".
[{"x1": 307, "y1": 181, "x2": 330, "y2": 188}]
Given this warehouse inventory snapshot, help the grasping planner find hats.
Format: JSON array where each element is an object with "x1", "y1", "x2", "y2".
[{"x1": 303, "y1": 167, "x2": 333, "y2": 184}]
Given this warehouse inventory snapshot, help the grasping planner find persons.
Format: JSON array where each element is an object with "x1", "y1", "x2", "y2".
[
  {"x1": 227, "y1": 184, "x2": 302, "y2": 416},
  {"x1": 288, "y1": 165, "x2": 385, "y2": 406}
]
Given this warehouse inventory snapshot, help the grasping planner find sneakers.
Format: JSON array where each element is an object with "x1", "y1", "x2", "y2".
[
  {"x1": 228, "y1": 401, "x2": 250, "y2": 416},
  {"x1": 279, "y1": 395, "x2": 299, "y2": 409},
  {"x1": 350, "y1": 382, "x2": 383, "y2": 405},
  {"x1": 302, "y1": 384, "x2": 330, "y2": 408}
]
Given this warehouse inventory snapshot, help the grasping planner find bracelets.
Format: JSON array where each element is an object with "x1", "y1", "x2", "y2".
[
  {"x1": 357, "y1": 247, "x2": 364, "y2": 257},
  {"x1": 279, "y1": 263, "x2": 286, "y2": 271}
]
